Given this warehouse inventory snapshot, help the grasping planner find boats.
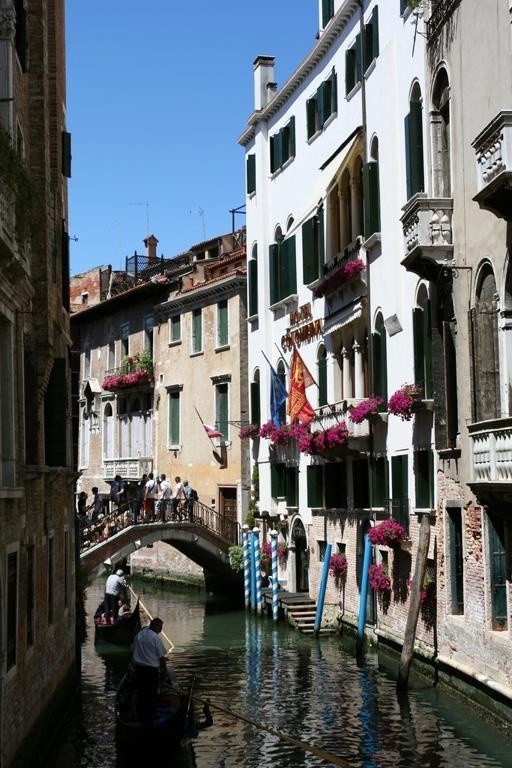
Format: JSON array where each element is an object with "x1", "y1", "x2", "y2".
[
  {"x1": 115, "y1": 664, "x2": 188, "y2": 748},
  {"x1": 93, "y1": 597, "x2": 141, "y2": 647}
]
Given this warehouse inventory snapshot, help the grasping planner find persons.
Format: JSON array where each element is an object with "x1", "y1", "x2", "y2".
[
  {"x1": 77, "y1": 472, "x2": 194, "y2": 548},
  {"x1": 94, "y1": 569, "x2": 134, "y2": 625},
  {"x1": 131, "y1": 617, "x2": 168, "y2": 725}
]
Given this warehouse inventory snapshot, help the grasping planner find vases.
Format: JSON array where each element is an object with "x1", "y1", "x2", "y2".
[
  {"x1": 322, "y1": 445, "x2": 344, "y2": 459},
  {"x1": 387, "y1": 539, "x2": 398, "y2": 547},
  {"x1": 410, "y1": 392, "x2": 422, "y2": 413}
]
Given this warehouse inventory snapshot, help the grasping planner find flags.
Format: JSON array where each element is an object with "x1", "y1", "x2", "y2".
[
  {"x1": 286, "y1": 346, "x2": 316, "y2": 429},
  {"x1": 270, "y1": 365, "x2": 288, "y2": 434}
]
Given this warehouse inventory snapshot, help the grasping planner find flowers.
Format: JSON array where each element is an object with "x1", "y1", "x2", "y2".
[
  {"x1": 313, "y1": 420, "x2": 349, "y2": 462},
  {"x1": 368, "y1": 519, "x2": 404, "y2": 546},
  {"x1": 387, "y1": 384, "x2": 422, "y2": 420},
  {"x1": 344, "y1": 259, "x2": 367, "y2": 277}
]
